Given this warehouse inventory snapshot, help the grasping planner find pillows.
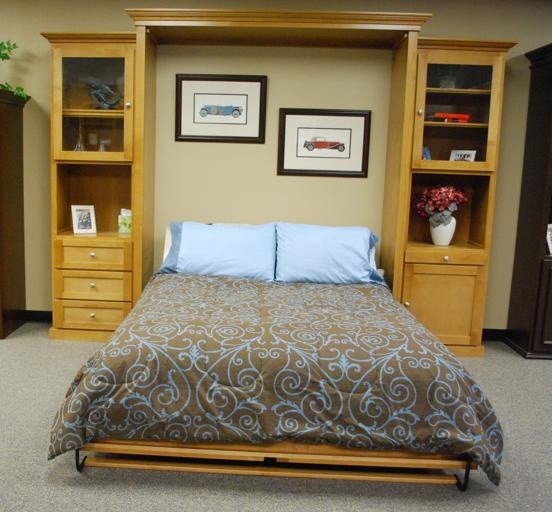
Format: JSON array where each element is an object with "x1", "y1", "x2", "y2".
[{"x1": 152, "y1": 220, "x2": 390, "y2": 295}]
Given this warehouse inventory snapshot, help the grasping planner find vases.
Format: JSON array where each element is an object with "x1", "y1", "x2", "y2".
[{"x1": 429, "y1": 217, "x2": 457, "y2": 246}]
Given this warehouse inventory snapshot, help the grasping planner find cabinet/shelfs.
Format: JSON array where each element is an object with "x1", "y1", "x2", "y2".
[
  {"x1": 394, "y1": 36, "x2": 519, "y2": 359},
  {"x1": 40, "y1": 31, "x2": 136, "y2": 340}
]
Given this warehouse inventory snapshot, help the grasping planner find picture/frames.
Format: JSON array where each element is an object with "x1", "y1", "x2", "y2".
[
  {"x1": 69, "y1": 204, "x2": 98, "y2": 235},
  {"x1": 174, "y1": 73, "x2": 371, "y2": 179}
]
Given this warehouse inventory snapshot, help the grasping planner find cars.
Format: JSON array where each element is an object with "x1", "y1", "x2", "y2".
[{"x1": 200, "y1": 103, "x2": 245, "y2": 120}]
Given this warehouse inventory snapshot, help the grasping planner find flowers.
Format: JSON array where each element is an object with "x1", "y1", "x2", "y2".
[{"x1": 416, "y1": 184, "x2": 469, "y2": 228}]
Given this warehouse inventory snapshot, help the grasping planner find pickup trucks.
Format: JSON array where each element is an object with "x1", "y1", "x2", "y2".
[{"x1": 303, "y1": 137, "x2": 345, "y2": 152}]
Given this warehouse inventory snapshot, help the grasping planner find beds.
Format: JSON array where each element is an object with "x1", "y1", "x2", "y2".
[{"x1": 72, "y1": 220, "x2": 480, "y2": 494}]
